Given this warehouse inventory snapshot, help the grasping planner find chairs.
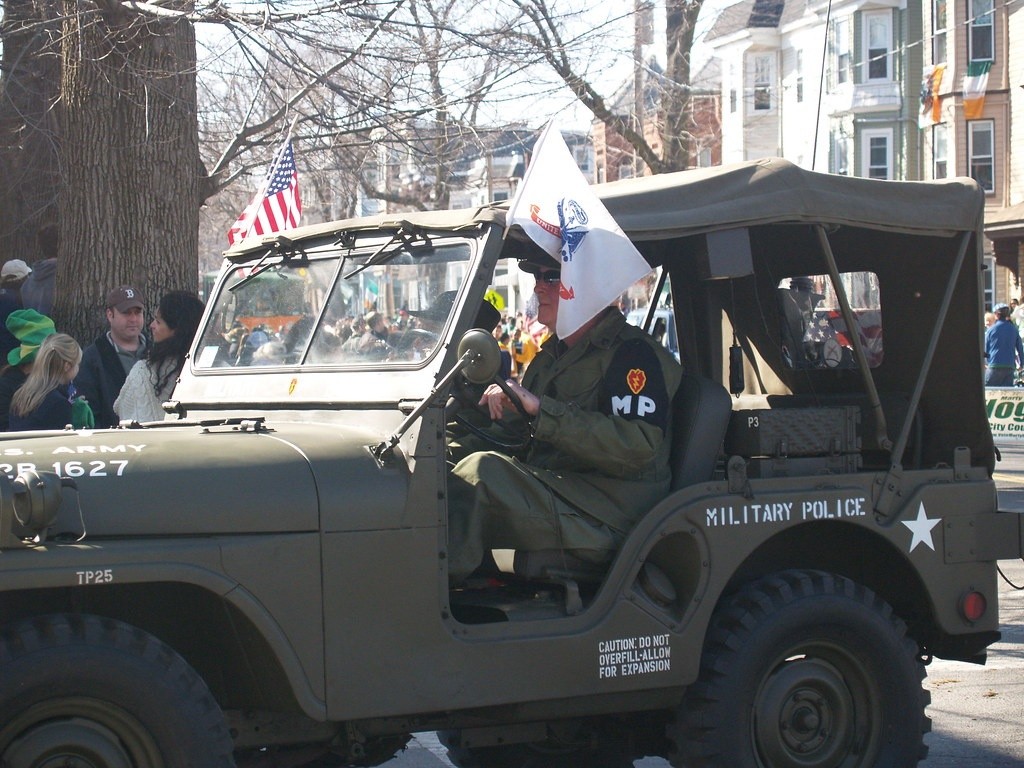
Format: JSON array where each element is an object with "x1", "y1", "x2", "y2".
[{"x1": 512, "y1": 371, "x2": 732, "y2": 603}]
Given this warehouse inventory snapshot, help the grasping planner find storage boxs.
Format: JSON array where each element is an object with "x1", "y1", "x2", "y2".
[{"x1": 723, "y1": 404, "x2": 862, "y2": 481}]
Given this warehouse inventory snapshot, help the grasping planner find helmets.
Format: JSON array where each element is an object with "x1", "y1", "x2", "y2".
[{"x1": 992, "y1": 303, "x2": 1009, "y2": 313}]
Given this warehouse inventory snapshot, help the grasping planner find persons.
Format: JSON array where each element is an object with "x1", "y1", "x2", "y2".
[
  {"x1": 208, "y1": 299, "x2": 546, "y2": 386},
  {"x1": 9, "y1": 334, "x2": 96, "y2": 429},
  {"x1": 396, "y1": 289, "x2": 535, "y2": 463},
  {"x1": 984, "y1": 297, "x2": 1024, "y2": 388},
  {"x1": 438, "y1": 243, "x2": 684, "y2": 600},
  {"x1": 0, "y1": 258, "x2": 206, "y2": 428}
]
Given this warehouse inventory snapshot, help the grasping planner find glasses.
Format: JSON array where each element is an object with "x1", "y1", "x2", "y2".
[{"x1": 534, "y1": 270, "x2": 560, "y2": 284}]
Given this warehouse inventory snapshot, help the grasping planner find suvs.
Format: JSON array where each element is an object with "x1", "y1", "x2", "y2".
[
  {"x1": 202, "y1": 267, "x2": 306, "y2": 344},
  {"x1": 0, "y1": 158, "x2": 1023, "y2": 767}
]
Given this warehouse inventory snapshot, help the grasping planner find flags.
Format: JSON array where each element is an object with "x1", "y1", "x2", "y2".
[
  {"x1": 962, "y1": 60, "x2": 992, "y2": 119},
  {"x1": 363, "y1": 278, "x2": 379, "y2": 312},
  {"x1": 503, "y1": 117, "x2": 652, "y2": 341},
  {"x1": 229, "y1": 139, "x2": 303, "y2": 279},
  {"x1": 918, "y1": 62, "x2": 947, "y2": 129},
  {"x1": 799, "y1": 307, "x2": 883, "y2": 369}
]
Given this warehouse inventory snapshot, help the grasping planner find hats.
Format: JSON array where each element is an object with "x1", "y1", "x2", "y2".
[
  {"x1": 107, "y1": 284, "x2": 147, "y2": 315},
  {"x1": 5, "y1": 308, "x2": 58, "y2": 366},
  {"x1": 405, "y1": 290, "x2": 501, "y2": 334},
  {"x1": 1, "y1": 259, "x2": 33, "y2": 282},
  {"x1": 518, "y1": 251, "x2": 561, "y2": 273}
]
